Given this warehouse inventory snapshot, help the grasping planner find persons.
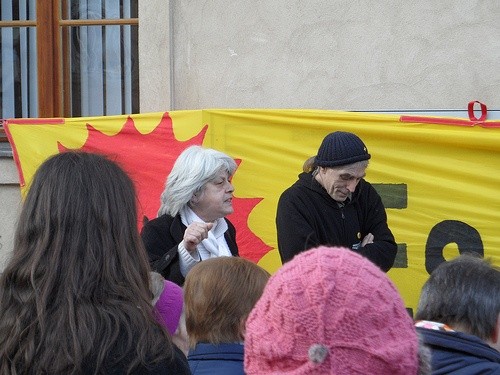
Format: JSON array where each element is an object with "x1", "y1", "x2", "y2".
[
  {"x1": 276, "y1": 131, "x2": 398, "y2": 273},
  {"x1": 148, "y1": 272, "x2": 191, "y2": 358},
  {"x1": 414, "y1": 254, "x2": 500, "y2": 375},
  {"x1": 0, "y1": 150, "x2": 191, "y2": 375},
  {"x1": 140, "y1": 145, "x2": 240, "y2": 287},
  {"x1": 243, "y1": 246, "x2": 431, "y2": 375},
  {"x1": 183, "y1": 256, "x2": 272, "y2": 375}
]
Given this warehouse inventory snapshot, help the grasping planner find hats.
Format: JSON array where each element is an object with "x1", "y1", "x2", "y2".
[
  {"x1": 314, "y1": 131, "x2": 371, "y2": 167},
  {"x1": 243, "y1": 245, "x2": 420, "y2": 375}
]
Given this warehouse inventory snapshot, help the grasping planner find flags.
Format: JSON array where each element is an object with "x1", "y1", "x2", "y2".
[{"x1": 2, "y1": 108, "x2": 500, "y2": 320}]
